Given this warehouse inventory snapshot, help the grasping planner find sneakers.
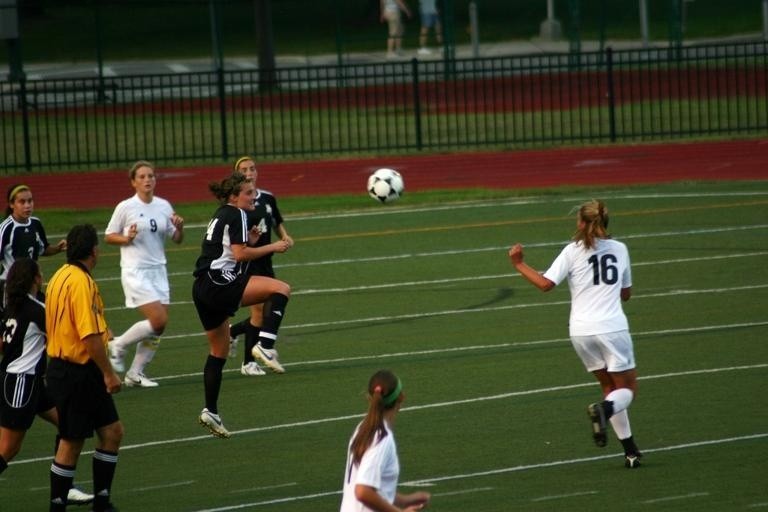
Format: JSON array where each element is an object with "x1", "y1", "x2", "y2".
[
  {"x1": 588, "y1": 403, "x2": 610, "y2": 448},
  {"x1": 199, "y1": 407, "x2": 232, "y2": 440},
  {"x1": 106, "y1": 338, "x2": 127, "y2": 373},
  {"x1": 124, "y1": 370, "x2": 159, "y2": 389},
  {"x1": 66, "y1": 489, "x2": 96, "y2": 506},
  {"x1": 624, "y1": 456, "x2": 642, "y2": 469},
  {"x1": 239, "y1": 341, "x2": 286, "y2": 376},
  {"x1": 228, "y1": 322, "x2": 239, "y2": 359},
  {"x1": 384, "y1": 47, "x2": 434, "y2": 61}
]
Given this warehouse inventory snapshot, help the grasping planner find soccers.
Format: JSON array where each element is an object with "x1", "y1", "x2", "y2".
[{"x1": 366, "y1": 168, "x2": 405, "y2": 203}]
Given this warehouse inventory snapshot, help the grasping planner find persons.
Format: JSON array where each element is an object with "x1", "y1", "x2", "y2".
[
  {"x1": 379, "y1": 0, "x2": 413, "y2": 60},
  {"x1": 417, "y1": 0, "x2": 448, "y2": 55},
  {"x1": 508, "y1": 200, "x2": 643, "y2": 469},
  {"x1": 339, "y1": 370, "x2": 431, "y2": 512}
]
